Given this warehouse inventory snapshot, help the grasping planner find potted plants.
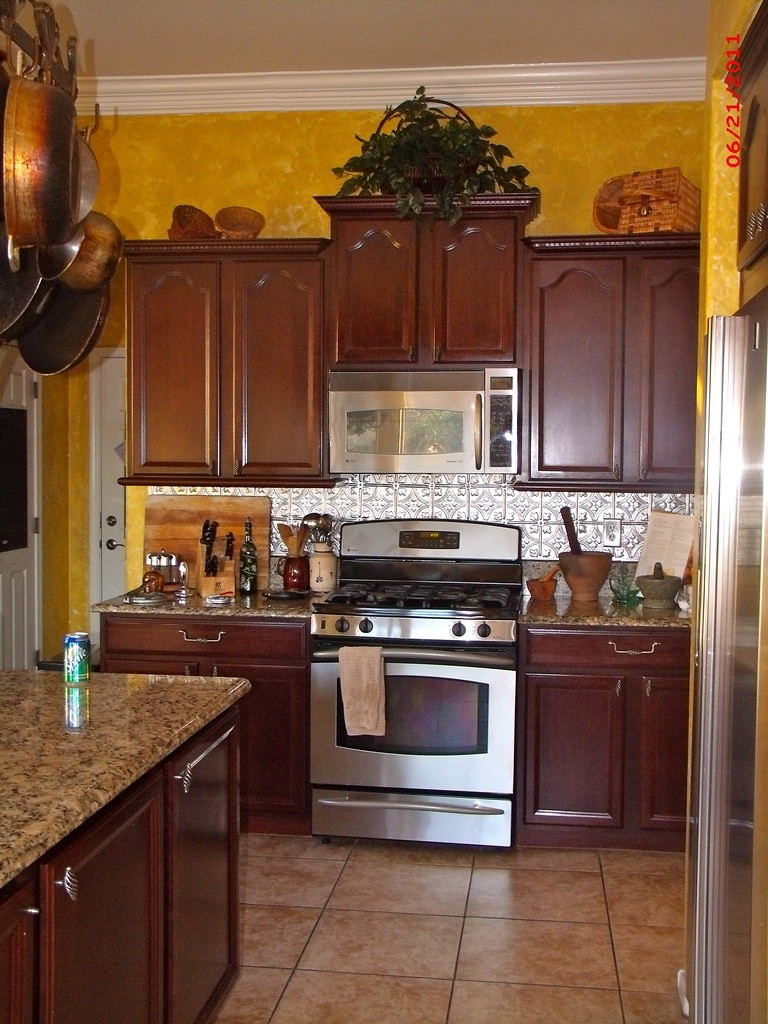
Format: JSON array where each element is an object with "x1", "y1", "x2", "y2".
[{"x1": 328, "y1": 85, "x2": 540, "y2": 227}]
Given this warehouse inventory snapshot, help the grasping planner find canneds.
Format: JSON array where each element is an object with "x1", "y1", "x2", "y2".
[
  {"x1": 64, "y1": 631, "x2": 90, "y2": 682},
  {"x1": 65, "y1": 683, "x2": 90, "y2": 734}
]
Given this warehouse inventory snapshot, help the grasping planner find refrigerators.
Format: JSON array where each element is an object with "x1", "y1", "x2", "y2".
[{"x1": 686, "y1": 286, "x2": 767, "y2": 1024}]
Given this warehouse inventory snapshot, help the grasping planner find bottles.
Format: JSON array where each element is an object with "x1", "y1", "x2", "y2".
[
  {"x1": 310, "y1": 541, "x2": 337, "y2": 592},
  {"x1": 240, "y1": 517, "x2": 257, "y2": 595}
]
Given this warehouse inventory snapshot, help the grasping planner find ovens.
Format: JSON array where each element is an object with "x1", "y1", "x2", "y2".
[{"x1": 310, "y1": 640, "x2": 516, "y2": 849}]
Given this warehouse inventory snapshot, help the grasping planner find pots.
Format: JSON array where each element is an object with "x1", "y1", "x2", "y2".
[{"x1": 0, "y1": 0, "x2": 122, "y2": 375}]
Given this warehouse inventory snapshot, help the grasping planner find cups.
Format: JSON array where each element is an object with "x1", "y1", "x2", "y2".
[{"x1": 277, "y1": 553, "x2": 309, "y2": 591}]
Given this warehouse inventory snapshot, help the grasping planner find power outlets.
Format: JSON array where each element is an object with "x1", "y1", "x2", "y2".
[{"x1": 603, "y1": 518, "x2": 621, "y2": 547}]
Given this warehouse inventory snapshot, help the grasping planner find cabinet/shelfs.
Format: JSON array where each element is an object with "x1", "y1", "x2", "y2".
[
  {"x1": 117, "y1": 194, "x2": 700, "y2": 492},
  {"x1": 100, "y1": 614, "x2": 311, "y2": 835},
  {"x1": 0, "y1": 703, "x2": 242, "y2": 1024},
  {"x1": 514, "y1": 626, "x2": 687, "y2": 852}
]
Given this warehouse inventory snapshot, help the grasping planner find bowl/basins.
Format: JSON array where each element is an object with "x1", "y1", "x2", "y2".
[
  {"x1": 610, "y1": 578, "x2": 641, "y2": 603},
  {"x1": 526, "y1": 579, "x2": 557, "y2": 599}
]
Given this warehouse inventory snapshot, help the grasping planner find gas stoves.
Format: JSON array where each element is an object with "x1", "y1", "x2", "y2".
[{"x1": 310, "y1": 519, "x2": 521, "y2": 642}]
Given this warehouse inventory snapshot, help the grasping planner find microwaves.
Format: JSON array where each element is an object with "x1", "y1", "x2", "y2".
[{"x1": 328, "y1": 368, "x2": 519, "y2": 474}]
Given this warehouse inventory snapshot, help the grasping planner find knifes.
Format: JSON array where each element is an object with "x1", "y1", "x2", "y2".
[{"x1": 194, "y1": 520, "x2": 236, "y2": 597}]
